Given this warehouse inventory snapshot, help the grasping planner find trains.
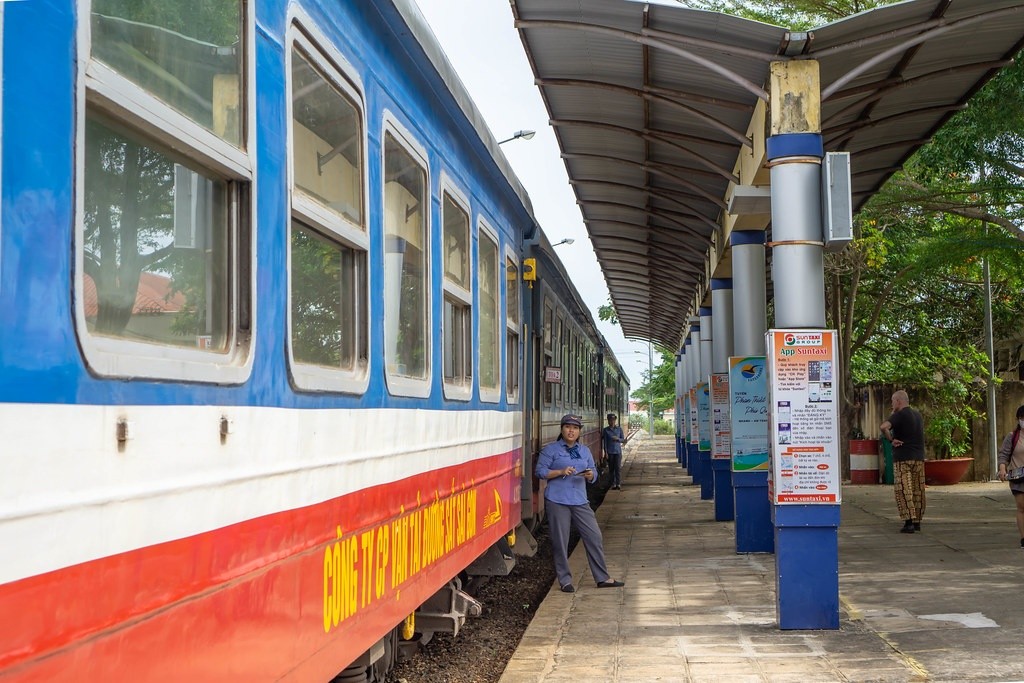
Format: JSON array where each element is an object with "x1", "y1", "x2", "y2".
[{"x1": 1, "y1": 0, "x2": 633, "y2": 683}]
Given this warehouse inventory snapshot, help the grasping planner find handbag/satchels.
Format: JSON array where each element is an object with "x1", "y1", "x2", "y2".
[{"x1": 1008, "y1": 468, "x2": 1024, "y2": 482}]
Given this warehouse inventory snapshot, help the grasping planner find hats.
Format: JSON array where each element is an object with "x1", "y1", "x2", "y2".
[
  {"x1": 1016, "y1": 405, "x2": 1024, "y2": 416},
  {"x1": 607, "y1": 414, "x2": 617, "y2": 418},
  {"x1": 561, "y1": 414, "x2": 584, "y2": 428}
]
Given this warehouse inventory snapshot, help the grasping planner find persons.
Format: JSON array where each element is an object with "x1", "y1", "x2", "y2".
[
  {"x1": 602, "y1": 412, "x2": 625, "y2": 491},
  {"x1": 997, "y1": 406, "x2": 1023, "y2": 548},
  {"x1": 879, "y1": 388, "x2": 928, "y2": 534},
  {"x1": 533, "y1": 414, "x2": 627, "y2": 592}
]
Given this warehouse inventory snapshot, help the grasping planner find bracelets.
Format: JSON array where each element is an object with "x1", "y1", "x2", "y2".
[{"x1": 890, "y1": 437, "x2": 894, "y2": 444}]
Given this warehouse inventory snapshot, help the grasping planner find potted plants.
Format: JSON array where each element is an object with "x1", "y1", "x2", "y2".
[{"x1": 907, "y1": 345, "x2": 1003, "y2": 486}]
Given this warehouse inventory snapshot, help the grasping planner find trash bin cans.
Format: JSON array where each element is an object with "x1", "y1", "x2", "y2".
[{"x1": 881, "y1": 434, "x2": 894, "y2": 484}]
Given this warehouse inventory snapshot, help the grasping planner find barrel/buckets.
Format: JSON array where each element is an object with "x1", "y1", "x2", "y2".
[{"x1": 849, "y1": 440, "x2": 879, "y2": 485}]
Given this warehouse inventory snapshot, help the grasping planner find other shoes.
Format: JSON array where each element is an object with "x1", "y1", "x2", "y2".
[
  {"x1": 561, "y1": 584, "x2": 574, "y2": 592},
  {"x1": 612, "y1": 485, "x2": 621, "y2": 490},
  {"x1": 900, "y1": 523, "x2": 921, "y2": 533},
  {"x1": 1020, "y1": 538, "x2": 1024, "y2": 548},
  {"x1": 597, "y1": 579, "x2": 625, "y2": 587}
]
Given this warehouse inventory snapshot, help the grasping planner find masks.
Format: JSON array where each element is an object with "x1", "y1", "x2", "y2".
[{"x1": 1019, "y1": 420, "x2": 1024, "y2": 428}]
